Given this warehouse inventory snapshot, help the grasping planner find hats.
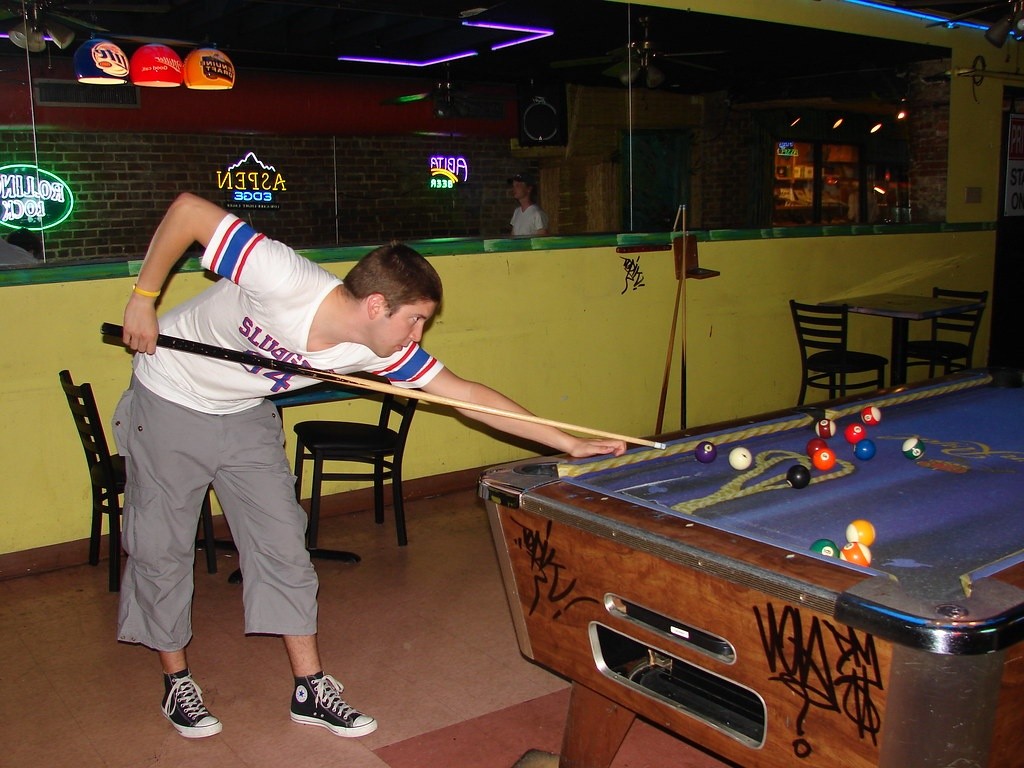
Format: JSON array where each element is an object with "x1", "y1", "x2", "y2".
[{"x1": 507, "y1": 172, "x2": 528, "y2": 184}]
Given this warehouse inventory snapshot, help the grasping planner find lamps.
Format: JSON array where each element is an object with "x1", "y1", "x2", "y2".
[
  {"x1": 8, "y1": 16, "x2": 76, "y2": 52},
  {"x1": 181, "y1": 44, "x2": 234, "y2": 90},
  {"x1": 984, "y1": 12, "x2": 1024, "y2": 49},
  {"x1": 862, "y1": 116, "x2": 883, "y2": 133},
  {"x1": 826, "y1": 114, "x2": 844, "y2": 128},
  {"x1": 433, "y1": 90, "x2": 456, "y2": 119},
  {"x1": 783, "y1": 113, "x2": 801, "y2": 127},
  {"x1": 129, "y1": 41, "x2": 183, "y2": 88},
  {"x1": 73, "y1": 39, "x2": 129, "y2": 85},
  {"x1": 616, "y1": 47, "x2": 675, "y2": 90}
]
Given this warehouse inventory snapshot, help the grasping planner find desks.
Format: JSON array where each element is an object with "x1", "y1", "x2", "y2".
[
  {"x1": 193, "y1": 378, "x2": 377, "y2": 584},
  {"x1": 476, "y1": 371, "x2": 1024, "y2": 768},
  {"x1": 818, "y1": 293, "x2": 985, "y2": 388}
]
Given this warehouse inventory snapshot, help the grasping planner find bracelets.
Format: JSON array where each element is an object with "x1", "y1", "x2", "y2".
[{"x1": 133, "y1": 285, "x2": 161, "y2": 297}]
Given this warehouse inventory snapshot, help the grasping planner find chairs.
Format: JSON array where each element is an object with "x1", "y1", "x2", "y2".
[
  {"x1": 294, "y1": 383, "x2": 422, "y2": 547},
  {"x1": 908, "y1": 287, "x2": 988, "y2": 379},
  {"x1": 789, "y1": 300, "x2": 889, "y2": 407},
  {"x1": 58, "y1": 370, "x2": 219, "y2": 593}
]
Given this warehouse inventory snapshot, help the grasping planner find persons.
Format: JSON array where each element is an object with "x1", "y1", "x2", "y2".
[
  {"x1": 506, "y1": 172, "x2": 549, "y2": 236},
  {"x1": 847, "y1": 179, "x2": 879, "y2": 224},
  {"x1": 0, "y1": 227, "x2": 44, "y2": 264},
  {"x1": 112, "y1": 191, "x2": 625, "y2": 738}
]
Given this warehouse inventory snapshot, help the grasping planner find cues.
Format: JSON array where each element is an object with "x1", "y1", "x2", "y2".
[
  {"x1": 655, "y1": 205, "x2": 687, "y2": 436},
  {"x1": 673, "y1": 205, "x2": 682, "y2": 231},
  {"x1": 100, "y1": 322, "x2": 667, "y2": 451}
]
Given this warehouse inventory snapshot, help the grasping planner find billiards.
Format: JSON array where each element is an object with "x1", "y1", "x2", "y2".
[
  {"x1": 901, "y1": 438, "x2": 926, "y2": 461},
  {"x1": 728, "y1": 447, "x2": 752, "y2": 471},
  {"x1": 844, "y1": 422, "x2": 866, "y2": 444},
  {"x1": 695, "y1": 441, "x2": 718, "y2": 464},
  {"x1": 786, "y1": 465, "x2": 811, "y2": 489},
  {"x1": 861, "y1": 406, "x2": 882, "y2": 426},
  {"x1": 809, "y1": 539, "x2": 839, "y2": 558},
  {"x1": 846, "y1": 520, "x2": 876, "y2": 548},
  {"x1": 815, "y1": 419, "x2": 837, "y2": 439},
  {"x1": 840, "y1": 542, "x2": 872, "y2": 567},
  {"x1": 807, "y1": 438, "x2": 836, "y2": 471},
  {"x1": 853, "y1": 439, "x2": 876, "y2": 461}
]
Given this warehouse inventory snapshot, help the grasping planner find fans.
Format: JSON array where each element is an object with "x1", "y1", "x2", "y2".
[
  {"x1": 548, "y1": 5, "x2": 729, "y2": 76},
  {"x1": 384, "y1": 64, "x2": 524, "y2": 109},
  {"x1": 924, "y1": 0, "x2": 1024, "y2": 28}
]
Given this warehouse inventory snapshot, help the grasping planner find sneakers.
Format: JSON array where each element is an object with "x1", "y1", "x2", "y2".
[
  {"x1": 161, "y1": 668, "x2": 222, "y2": 739},
  {"x1": 289, "y1": 670, "x2": 377, "y2": 737}
]
